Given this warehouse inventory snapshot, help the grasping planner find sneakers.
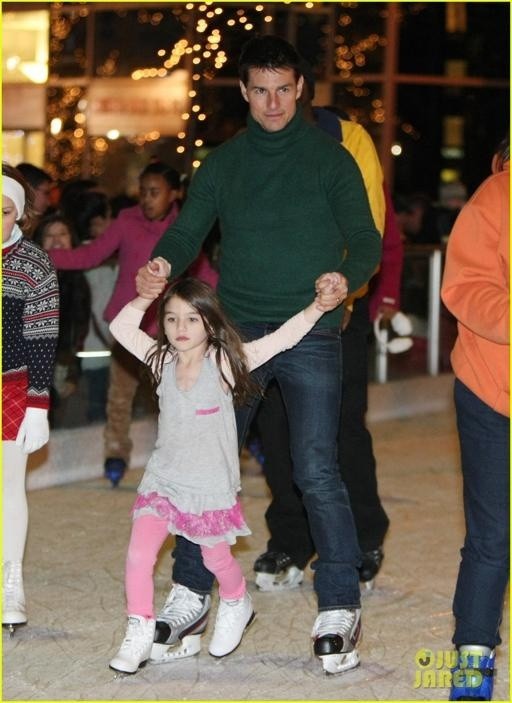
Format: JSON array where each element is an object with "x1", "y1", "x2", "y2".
[{"x1": 4, "y1": 558, "x2": 28, "y2": 625}]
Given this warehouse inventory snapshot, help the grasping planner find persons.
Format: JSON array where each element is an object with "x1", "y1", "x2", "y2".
[
  {"x1": 438, "y1": 140, "x2": 511, "y2": 698},
  {"x1": 2, "y1": 31, "x2": 405, "y2": 679}
]
